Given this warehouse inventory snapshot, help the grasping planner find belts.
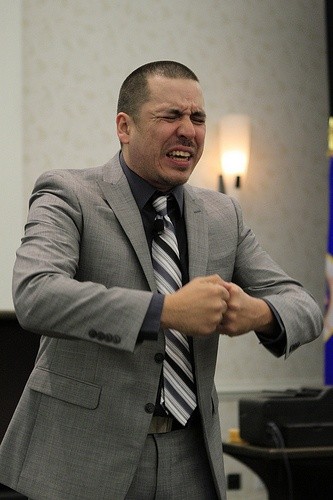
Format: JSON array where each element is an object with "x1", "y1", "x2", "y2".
[{"x1": 148, "y1": 414, "x2": 175, "y2": 434}]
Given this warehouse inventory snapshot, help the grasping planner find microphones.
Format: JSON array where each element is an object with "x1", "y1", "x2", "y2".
[{"x1": 153, "y1": 219, "x2": 164, "y2": 236}]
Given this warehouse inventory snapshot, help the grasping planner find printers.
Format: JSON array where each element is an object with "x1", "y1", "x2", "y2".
[{"x1": 239, "y1": 386, "x2": 333, "y2": 448}]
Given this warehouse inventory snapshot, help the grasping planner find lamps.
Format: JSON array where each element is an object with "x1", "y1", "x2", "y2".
[{"x1": 221, "y1": 113, "x2": 251, "y2": 187}]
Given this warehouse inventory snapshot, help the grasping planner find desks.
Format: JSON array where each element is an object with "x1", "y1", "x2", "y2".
[{"x1": 222, "y1": 441, "x2": 333, "y2": 500}]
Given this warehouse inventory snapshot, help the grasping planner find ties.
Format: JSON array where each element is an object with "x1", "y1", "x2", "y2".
[{"x1": 151, "y1": 194, "x2": 198, "y2": 426}]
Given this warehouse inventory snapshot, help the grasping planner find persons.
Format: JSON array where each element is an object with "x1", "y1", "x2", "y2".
[{"x1": 0, "y1": 61, "x2": 324, "y2": 500}]
